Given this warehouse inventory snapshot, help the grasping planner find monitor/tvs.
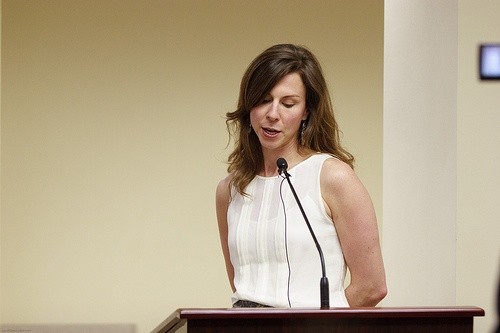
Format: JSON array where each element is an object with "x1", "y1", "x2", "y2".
[{"x1": 479, "y1": 44, "x2": 500, "y2": 80}]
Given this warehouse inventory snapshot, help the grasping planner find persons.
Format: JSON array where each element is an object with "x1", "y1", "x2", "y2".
[{"x1": 215, "y1": 43, "x2": 388, "y2": 311}]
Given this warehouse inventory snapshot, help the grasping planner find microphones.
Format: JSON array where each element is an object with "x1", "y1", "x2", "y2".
[{"x1": 277, "y1": 157, "x2": 329, "y2": 310}]
